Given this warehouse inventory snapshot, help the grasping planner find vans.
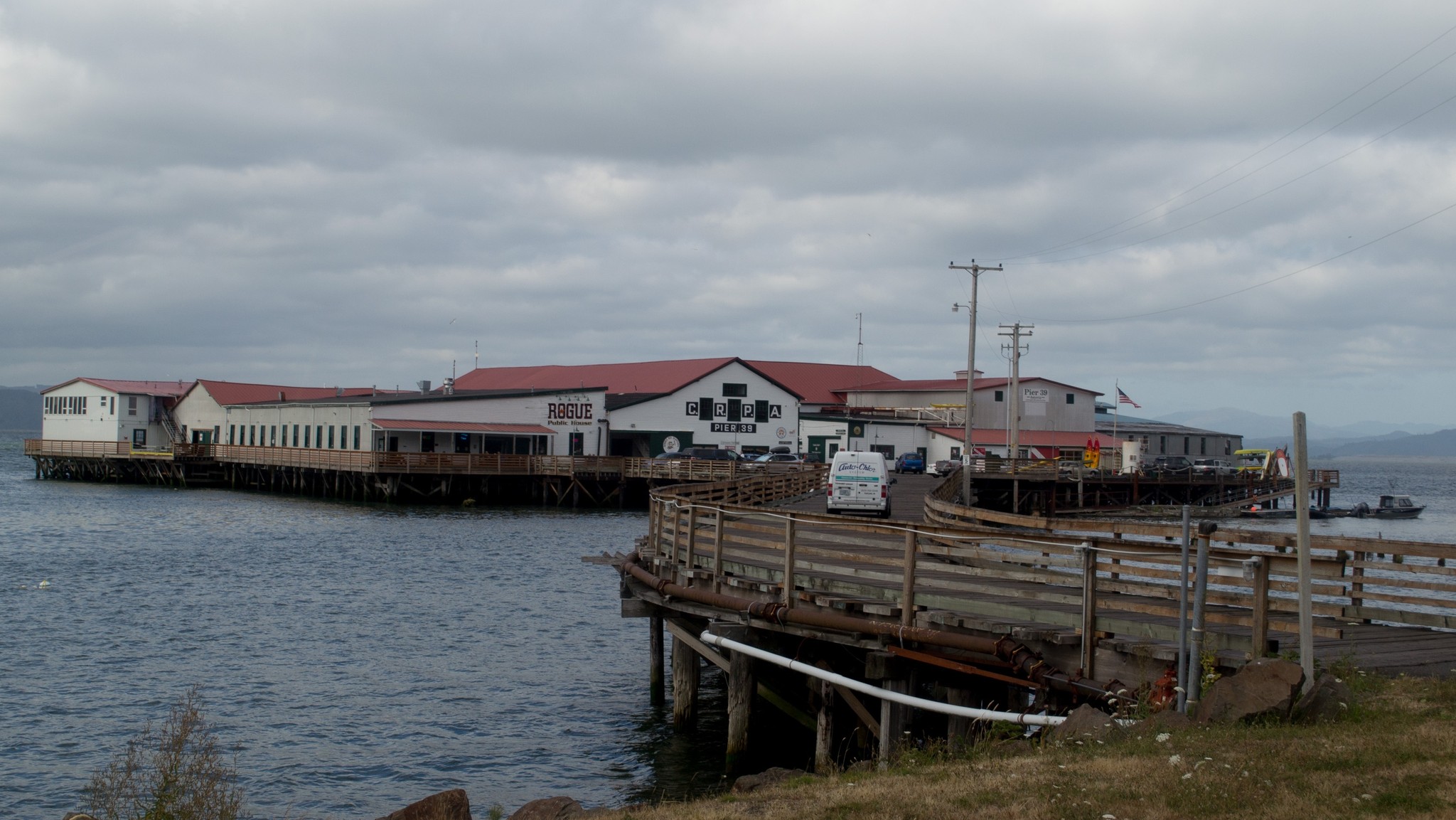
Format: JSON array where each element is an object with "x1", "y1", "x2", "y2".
[
  {"x1": 828, "y1": 451, "x2": 892, "y2": 518},
  {"x1": 682, "y1": 448, "x2": 753, "y2": 473}
]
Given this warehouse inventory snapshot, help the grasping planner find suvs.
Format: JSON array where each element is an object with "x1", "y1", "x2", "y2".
[
  {"x1": 1192, "y1": 458, "x2": 1239, "y2": 478},
  {"x1": 740, "y1": 446, "x2": 804, "y2": 473},
  {"x1": 1153, "y1": 457, "x2": 1194, "y2": 478},
  {"x1": 798, "y1": 452, "x2": 821, "y2": 464}
]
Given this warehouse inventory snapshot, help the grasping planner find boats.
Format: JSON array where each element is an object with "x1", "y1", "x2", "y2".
[{"x1": 1357, "y1": 495, "x2": 1427, "y2": 519}]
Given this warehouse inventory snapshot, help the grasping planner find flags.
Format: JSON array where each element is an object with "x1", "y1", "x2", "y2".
[{"x1": 1116, "y1": 387, "x2": 1141, "y2": 408}]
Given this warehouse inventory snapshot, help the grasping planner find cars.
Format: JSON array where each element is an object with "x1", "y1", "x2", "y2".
[
  {"x1": 740, "y1": 454, "x2": 761, "y2": 462},
  {"x1": 926, "y1": 455, "x2": 1004, "y2": 478},
  {"x1": 1048, "y1": 461, "x2": 1100, "y2": 478},
  {"x1": 894, "y1": 452, "x2": 924, "y2": 475},
  {"x1": 643, "y1": 452, "x2": 692, "y2": 475}
]
